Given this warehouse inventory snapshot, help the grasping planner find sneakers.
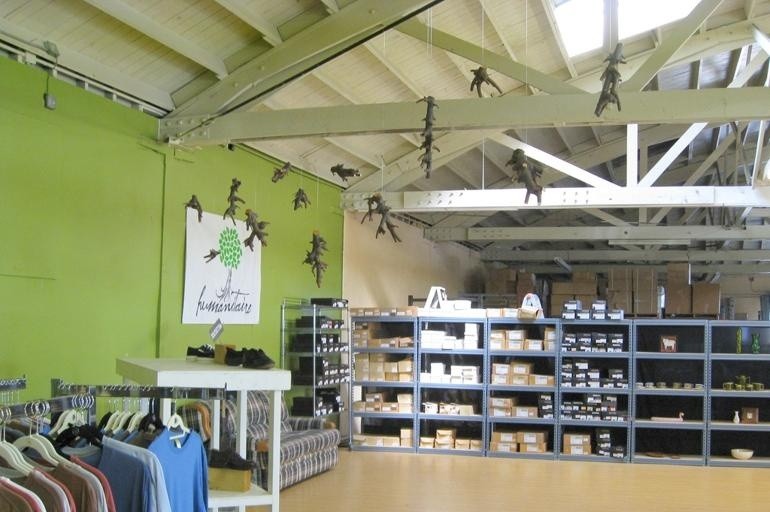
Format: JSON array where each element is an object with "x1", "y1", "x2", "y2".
[{"x1": 186, "y1": 344, "x2": 275, "y2": 369}]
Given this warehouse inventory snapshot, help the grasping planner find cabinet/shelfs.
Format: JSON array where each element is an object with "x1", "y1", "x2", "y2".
[
  {"x1": 417, "y1": 316, "x2": 488, "y2": 459},
  {"x1": 558, "y1": 318, "x2": 633, "y2": 464},
  {"x1": 634, "y1": 318, "x2": 708, "y2": 468},
  {"x1": 351, "y1": 316, "x2": 417, "y2": 454},
  {"x1": 707, "y1": 319, "x2": 770, "y2": 468},
  {"x1": 488, "y1": 318, "x2": 560, "y2": 462},
  {"x1": 282, "y1": 301, "x2": 351, "y2": 452}
]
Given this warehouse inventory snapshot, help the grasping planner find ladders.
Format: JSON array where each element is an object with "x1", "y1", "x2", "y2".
[
  {"x1": 417, "y1": 286, "x2": 467, "y2": 404},
  {"x1": 505, "y1": 291, "x2": 556, "y2": 366}
]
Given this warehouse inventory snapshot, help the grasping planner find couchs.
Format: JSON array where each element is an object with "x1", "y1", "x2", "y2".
[{"x1": 179, "y1": 391, "x2": 339, "y2": 492}]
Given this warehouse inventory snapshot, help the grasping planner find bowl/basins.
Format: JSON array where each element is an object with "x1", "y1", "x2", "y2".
[{"x1": 730, "y1": 448, "x2": 754, "y2": 460}]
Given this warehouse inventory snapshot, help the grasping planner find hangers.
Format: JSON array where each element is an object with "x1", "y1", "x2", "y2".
[{"x1": 1, "y1": 377, "x2": 189, "y2": 479}]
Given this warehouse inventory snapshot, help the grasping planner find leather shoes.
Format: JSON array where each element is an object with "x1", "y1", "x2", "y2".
[{"x1": 208, "y1": 448, "x2": 256, "y2": 469}]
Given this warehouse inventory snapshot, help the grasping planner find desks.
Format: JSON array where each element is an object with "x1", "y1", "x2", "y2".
[{"x1": 118, "y1": 355, "x2": 292, "y2": 511}]
